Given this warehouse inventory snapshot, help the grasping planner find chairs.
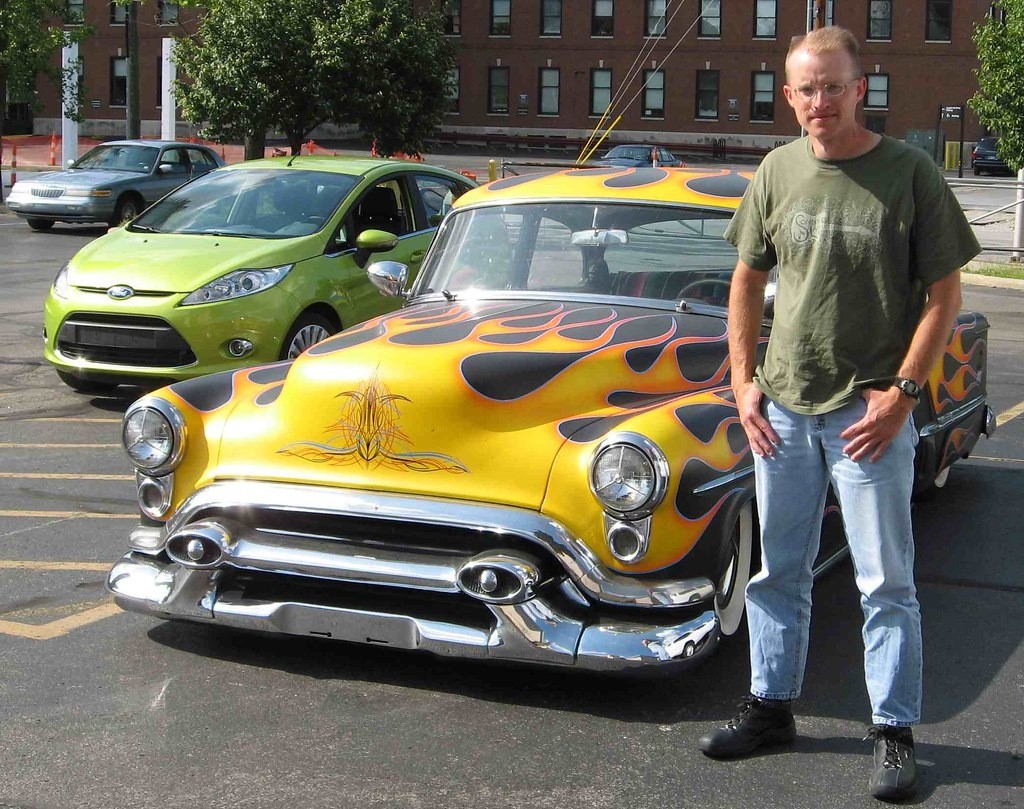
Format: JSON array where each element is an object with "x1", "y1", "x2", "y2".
[
  {"x1": 352, "y1": 185, "x2": 401, "y2": 237},
  {"x1": 252, "y1": 180, "x2": 311, "y2": 234}
]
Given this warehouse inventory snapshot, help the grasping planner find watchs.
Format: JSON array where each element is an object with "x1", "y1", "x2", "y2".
[{"x1": 893, "y1": 377, "x2": 922, "y2": 400}]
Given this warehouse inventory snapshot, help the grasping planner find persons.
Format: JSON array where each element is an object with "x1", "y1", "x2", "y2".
[{"x1": 698, "y1": 25, "x2": 983, "y2": 801}]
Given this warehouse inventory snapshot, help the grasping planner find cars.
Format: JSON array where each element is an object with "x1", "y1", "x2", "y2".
[
  {"x1": 5, "y1": 138, "x2": 228, "y2": 231},
  {"x1": 591, "y1": 144, "x2": 687, "y2": 169},
  {"x1": 43, "y1": 151, "x2": 514, "y2": 395}
]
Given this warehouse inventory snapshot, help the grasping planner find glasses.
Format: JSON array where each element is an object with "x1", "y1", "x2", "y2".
[{"x1": 786, "y1": 77, "x2": 863, "y2": 100}]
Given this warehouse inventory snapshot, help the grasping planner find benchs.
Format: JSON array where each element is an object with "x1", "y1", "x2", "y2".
[{"x1": 604, "y1": 268, "x2": 735, "y2": 308}]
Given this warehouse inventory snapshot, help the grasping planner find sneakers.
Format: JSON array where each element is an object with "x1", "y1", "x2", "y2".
[
  {"x1": 860, "y1": 723, "x2": 921, "y2": 799},
  {"x1": 695, "y1": 691, "x2": 797, "y2": 757}
]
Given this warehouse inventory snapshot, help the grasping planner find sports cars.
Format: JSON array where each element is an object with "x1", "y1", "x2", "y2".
[{"x1": 106, "y1": 146, "x2": 1000, "y2": 683}]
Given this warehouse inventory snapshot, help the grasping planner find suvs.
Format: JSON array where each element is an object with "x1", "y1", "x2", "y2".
[{"x1": 970, "y1": 136, "x2": 1016, "y2": 177}]
